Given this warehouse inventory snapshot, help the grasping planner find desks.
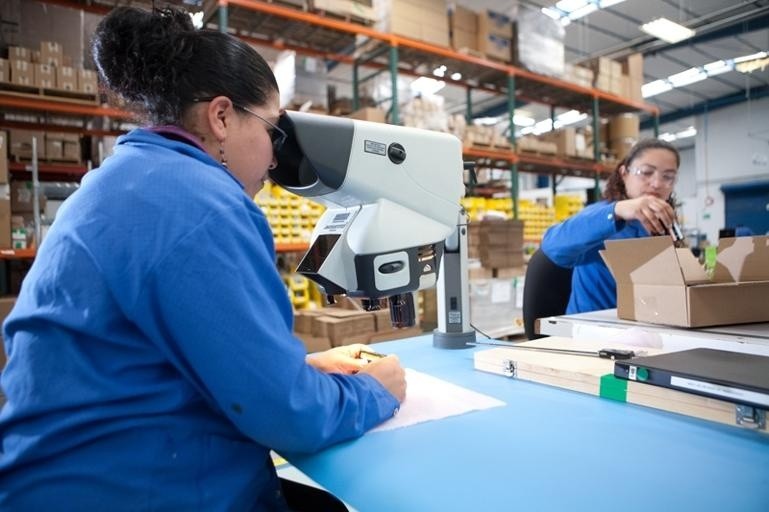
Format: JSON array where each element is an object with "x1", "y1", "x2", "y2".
[{"x1": 276, "y1": 330, "x2": 769, "y2": 512}]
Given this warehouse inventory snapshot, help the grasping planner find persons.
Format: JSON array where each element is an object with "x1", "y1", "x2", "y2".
[
  {"x1": 0, "y1": 3, "x2": 408, "y2": 508},
  {"x1": 541, "y1": 137, "x2": 683, "y2": 315}
]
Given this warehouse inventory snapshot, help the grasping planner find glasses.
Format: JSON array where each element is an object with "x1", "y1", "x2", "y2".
[
  {"x1": 627, "y1": 165, "x2": 675, "y2": 187},
  {"x1": 187, "y1": 95, "x2": 288, "y2": 152}
]
